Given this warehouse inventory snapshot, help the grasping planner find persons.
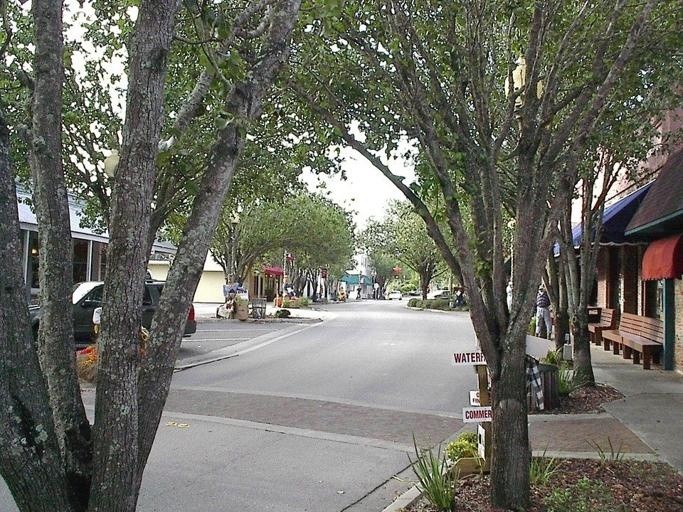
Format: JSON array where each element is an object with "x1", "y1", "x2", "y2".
[{"x1": 535, "y1": 285, "x2": 553, "y2": 339}]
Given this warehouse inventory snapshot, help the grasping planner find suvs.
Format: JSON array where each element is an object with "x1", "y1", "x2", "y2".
[{"x1": 26, "y1": 280, "x2": 197, "y2": 347}]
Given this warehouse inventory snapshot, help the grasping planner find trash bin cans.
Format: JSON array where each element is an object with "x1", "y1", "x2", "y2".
[{"x1": 252, "y1": 298, "x2": 266, "y2": 319}]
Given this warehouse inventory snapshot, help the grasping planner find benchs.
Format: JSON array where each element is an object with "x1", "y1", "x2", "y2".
[{"x1": 588, "y1": 306, "x2": 665, "y2": 371}]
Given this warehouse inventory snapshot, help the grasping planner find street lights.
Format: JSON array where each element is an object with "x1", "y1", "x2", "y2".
[
  {"x1": 280, "y1": 248, "x2": 291, "y2": 307},
  {"x1": 227, "y1": 210, "x2": 239, "y2": 285},
  {"x1": 103, "y1": 148, "x2": 120, "y2": 207},
  {"x1": 506, "y1": 221, "x2": 516, "y2": 310}
]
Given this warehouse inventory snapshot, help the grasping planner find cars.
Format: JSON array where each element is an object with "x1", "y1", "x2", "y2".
[
  {"x1": 388, "y1": 291, "x2": 402, "y2": 300},
  {"x1": 409, "y1": 288, "x2": 420, "y2": 295}
]
